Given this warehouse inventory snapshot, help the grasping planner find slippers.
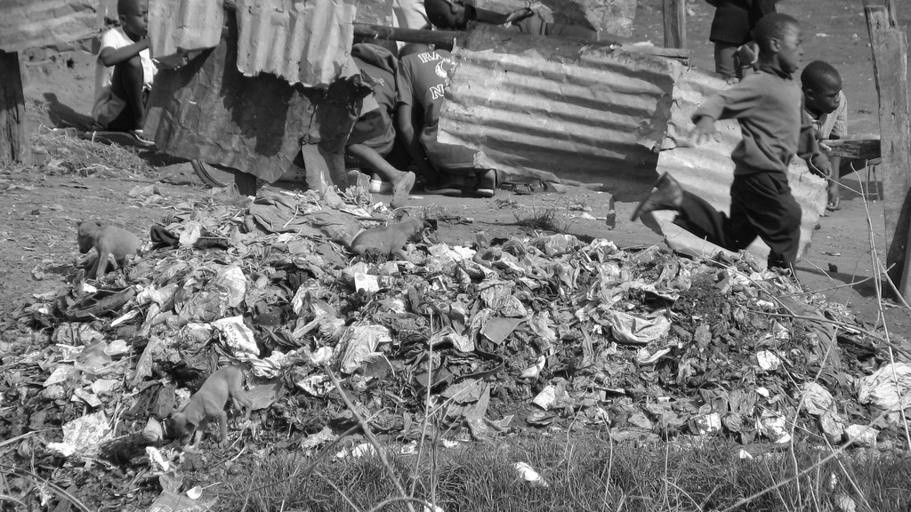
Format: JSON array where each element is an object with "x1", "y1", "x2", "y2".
[
  {"x1": 391, "y1": 171, "x2": 416, "y2": 208},
  {"x1": 467, "y1": 182, "x2": 494, "y2": 198},
  {"x1": 125, "y1": 117, "x2": 155, "y2": 148},
  {"x1": 368, "y1": 178, "x2": 390, "y2": 192},
  {"x1": 425, "y1": 182, "x2": 463, "y2": 197}
]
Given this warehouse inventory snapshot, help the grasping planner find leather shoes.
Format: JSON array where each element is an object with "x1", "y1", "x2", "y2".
[{"x1": 632, "y1": 172, "x2": 683, "y2": 221}]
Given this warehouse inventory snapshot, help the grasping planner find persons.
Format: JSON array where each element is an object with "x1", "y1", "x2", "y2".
[
  {"x1": 395, "y1": 44, "x2": 497, "y2": 196},
  {"x1": 708, "y1": 0, "x2": 776, "y2": 78},
  {"x1": 92, "y1": 0, "x2": 157, "y2": 130},
  {"x1": 631, "y1": 14, "x2": 831, "y2": 279},
  {"x1": 344, "y1": 55, "x2": 416, "y2": 208},
  {"x1": 789, "y1": 60, "x2": 849, "y2": 211}
]
